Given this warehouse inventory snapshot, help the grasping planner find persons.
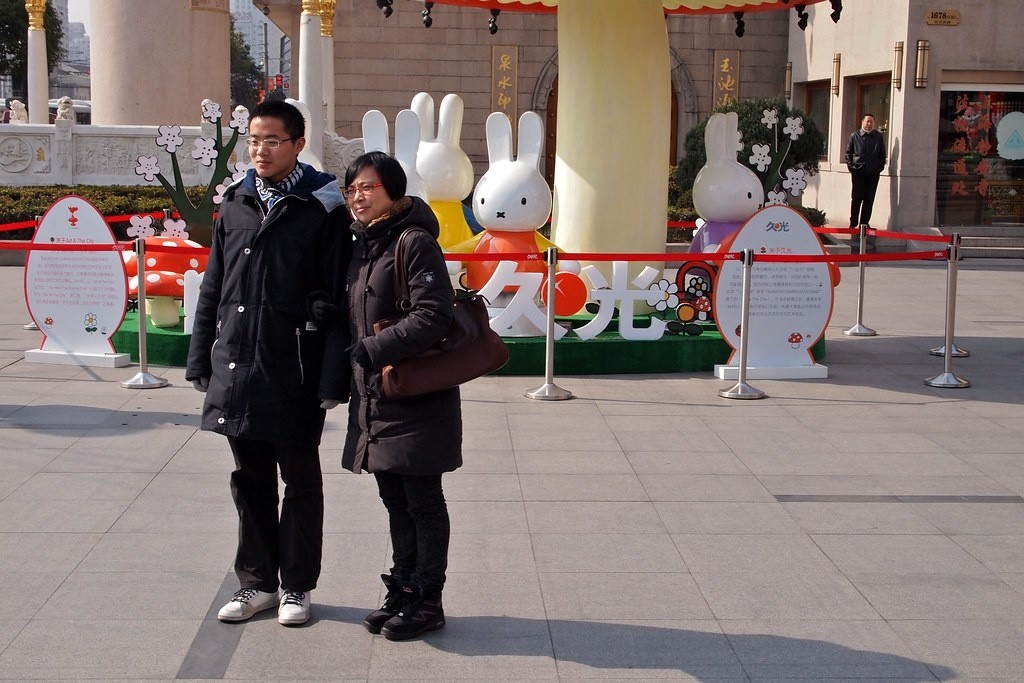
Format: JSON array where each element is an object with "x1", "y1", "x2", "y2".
[
  {"x1": 845, "y1": 114, "x2": 886, "y2": 229},
  {"x1": 185, "y1": 101, "x2": 349, "y2": 627},
  {"x1": 341, "y1": 151, "x2": 464, "y2": 641}
]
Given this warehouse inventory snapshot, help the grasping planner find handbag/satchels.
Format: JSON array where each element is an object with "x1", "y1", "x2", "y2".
[{"x1": 372, "y1": 226, "x2": 508, "y2": 397}]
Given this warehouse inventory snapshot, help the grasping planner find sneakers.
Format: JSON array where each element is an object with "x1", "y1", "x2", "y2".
[
  {"x1": 218, "y1": 586, "x2": 281, "y2": 621},
  {"x1": 278, "y1": 588, "x2": 311, "y2": 624}
]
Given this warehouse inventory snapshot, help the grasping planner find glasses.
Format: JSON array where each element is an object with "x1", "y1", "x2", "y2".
[
  {"x1": 246, "y1": 137, "x2": 292, "y2": 148},
  {"x1": 344, "y1": 183, "x2": 382, "y2": 197}
]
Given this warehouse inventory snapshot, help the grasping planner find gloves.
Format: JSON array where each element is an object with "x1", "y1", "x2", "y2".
[
  {"x1": 192, "y1": 377, "x2": 208, "y2": 392},
  {"x1": 320, "y1": 398, "x2": 342, "y2": 409},
  {"x1": 343, "y1": 342, "x2": 371, "y2": 368},
  {"x1": 312, "y1": 300, "x2": 337, "y2": 327}
]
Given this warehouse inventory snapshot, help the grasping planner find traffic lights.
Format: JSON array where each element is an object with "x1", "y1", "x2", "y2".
[
  {"x1": 284, "y1": 83, "x2": 289, "y2": 88},
  {"x1": 275, "y1": 73, "x2": 282, "y2": 85}
]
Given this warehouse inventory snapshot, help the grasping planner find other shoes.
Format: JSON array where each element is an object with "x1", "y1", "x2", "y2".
[
  {"x1": 849, "y1": 223, "x2": 855, "y2": 228},
  {"x1": 859, "y1": 224, "x2": 877, "y2": 230}
]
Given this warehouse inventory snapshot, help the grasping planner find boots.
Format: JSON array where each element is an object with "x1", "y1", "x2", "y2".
[
  {"x1": 380, "y1": 581, "x2": 446, "y2": 639},
  {"x1": 364, "y1": 573, "x2": 418, "y2": 633}
]
